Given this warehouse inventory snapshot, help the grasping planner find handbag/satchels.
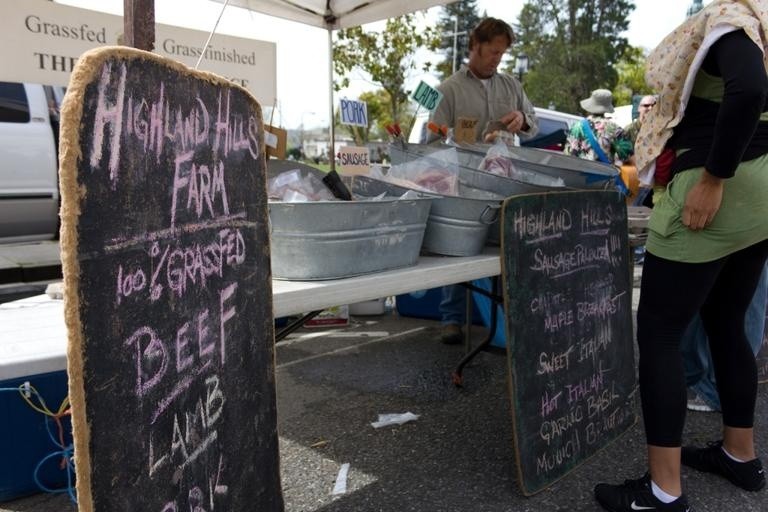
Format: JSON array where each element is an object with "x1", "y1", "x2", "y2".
[{"x1": 620, "y1": 146, "x2": 676, "y2": 206}]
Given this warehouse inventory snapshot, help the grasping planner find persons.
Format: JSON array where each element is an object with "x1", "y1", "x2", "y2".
[
  {"x1": 687, "y1": 251, "x2": 768, "y2": 413},
  {"x1": 425, "y1": 17, "x2": 540, "y2": 343},
  {"x1": 593, "y1": 0, "x2": 768, "y2": 511},
  {"x1": 563, "y1": 88, "x2": 624, "y2": 163},
  {"x1": 625, "y1": 95, "x2": 657, "y2": 146}
]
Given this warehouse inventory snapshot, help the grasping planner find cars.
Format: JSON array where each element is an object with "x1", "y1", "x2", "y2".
[{"x1": 0, "y1": 81, "x2": 66, "y2": 282}]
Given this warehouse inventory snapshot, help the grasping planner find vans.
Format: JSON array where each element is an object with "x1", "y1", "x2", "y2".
[{"x1": 408, "y1": 105, "x2": 586, "y2": 153}]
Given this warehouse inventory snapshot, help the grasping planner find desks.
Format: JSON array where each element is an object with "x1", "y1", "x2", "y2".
[{"x1": 2, "y1": 244, "x2": 502, "y2": 386}]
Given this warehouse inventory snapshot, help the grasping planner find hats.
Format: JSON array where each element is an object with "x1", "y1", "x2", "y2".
[{"x1": 580, "y1": 89, "x2": 615, "y2": 113}]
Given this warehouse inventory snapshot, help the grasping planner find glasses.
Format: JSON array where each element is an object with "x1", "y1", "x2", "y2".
[{"x1": 640, "y1": 104, "x2": 654, "y2": 107}]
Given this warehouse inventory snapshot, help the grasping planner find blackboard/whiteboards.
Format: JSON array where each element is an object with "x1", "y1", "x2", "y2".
[
  {"x1": 500, "y1": 188, "x2": 639, "y2": 500},
  {"x1": 57, "y1": 45, "x2": 285, "y2": 512}
]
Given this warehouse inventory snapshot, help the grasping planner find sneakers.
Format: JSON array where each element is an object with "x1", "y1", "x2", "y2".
[
  {"x1": 594, "y1": 470, "x2": 691, "y2": 512},
  {"x1": 687, "y1": 393, "x2": 715, "y2": 412},
  {"x1": 441, "y1": 323, "x2": 463, "y2": 344},
  {"x1": 680, "y1": 441, "x2": 766, "y2": 491}
]
